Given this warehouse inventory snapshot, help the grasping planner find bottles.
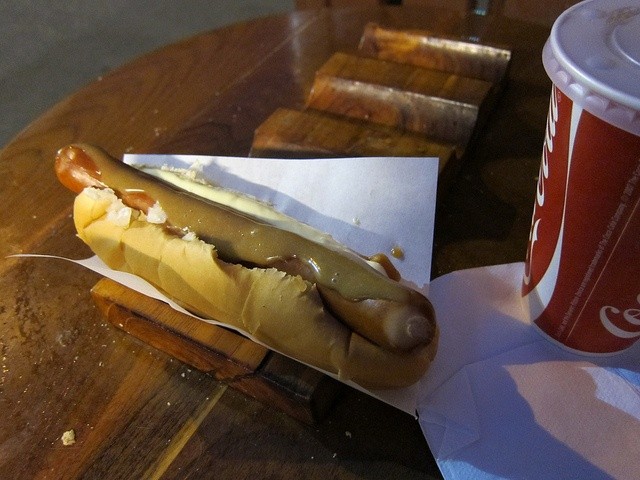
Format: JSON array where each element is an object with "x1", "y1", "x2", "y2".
[{"x1": 521, "y1": 1, "x2": 640, "y2": 358}]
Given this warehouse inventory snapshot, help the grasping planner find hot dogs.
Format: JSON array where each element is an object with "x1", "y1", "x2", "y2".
[{"x1": 52, "y1": 140, "x2": 440, "y2": 390}]
